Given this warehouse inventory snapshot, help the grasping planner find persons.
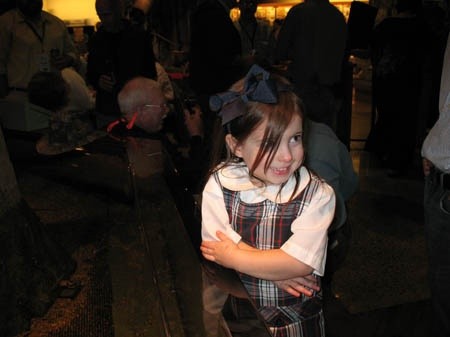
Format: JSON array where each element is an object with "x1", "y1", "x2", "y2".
[
  {"x1": 296, "y1": 83, "x2": 358, "y2": 304},
  {"x1": 276, "y1": 0, "x2": 354, "y2": 153},
  {"x1": 199, "y1": 64, "x2": 336, "y2": 337},
  {"x1": 421, "y1": 31, "x2": 450, "y2": 337},
  {"x1": 0, "y1": 0, "x2": 284, "y2": 263},
  {"x1": 347, "y1": 0, "x2": 450, "y2": 178}
]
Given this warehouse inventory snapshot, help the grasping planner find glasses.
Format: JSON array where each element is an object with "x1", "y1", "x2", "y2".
[{"x1": 141, "y1": 103, "x2": 175, "y2": 113}]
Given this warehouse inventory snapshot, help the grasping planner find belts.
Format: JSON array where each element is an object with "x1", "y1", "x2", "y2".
[
  {"x1": 10, "y1": 87, "x2": 29, "y2": 92},
  {"x1": 429, "y1": 166, "x2": 450, "y2": 190}
]
